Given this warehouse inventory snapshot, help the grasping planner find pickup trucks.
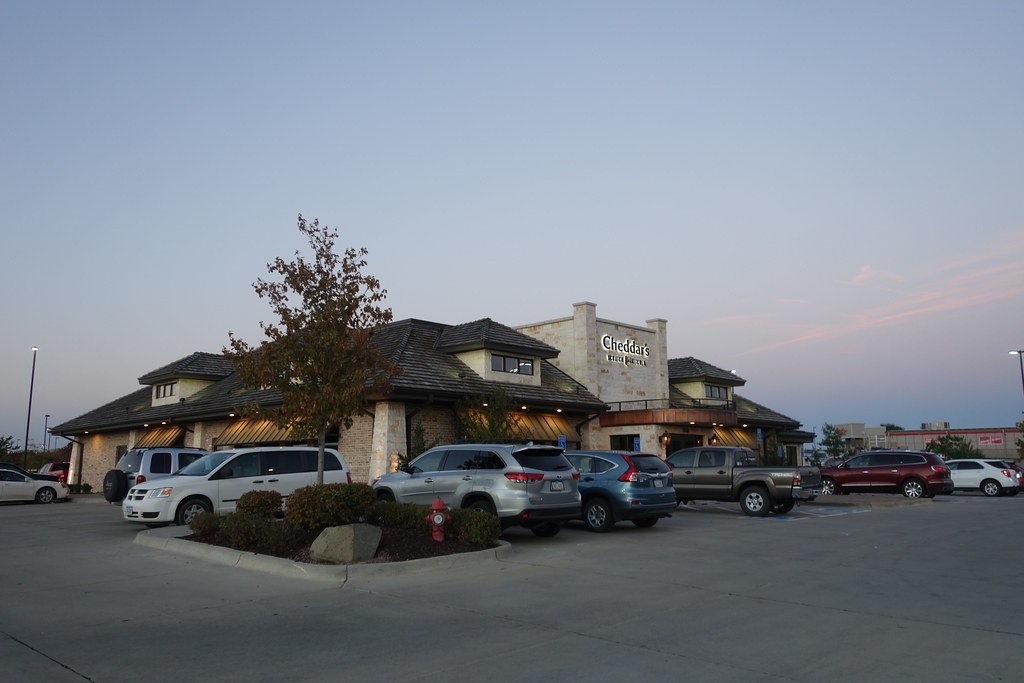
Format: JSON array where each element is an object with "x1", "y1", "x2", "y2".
[{"x1": 662, "y1": 442, "x2": 821, "y2": 517}]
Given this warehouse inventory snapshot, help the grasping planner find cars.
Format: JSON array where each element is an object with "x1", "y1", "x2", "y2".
[
  {"x1": 944, "y1": 459, "x2": 1019, "y2": 495},
  {"x1": 1004, "y1": 460, "x2": 1024, "y2": 496},
  {"x1": 816, "y1": 448, "x2": 954, "y2": 500},
  {"x1": 558, "y1": 450, "x2": 678, "y2": 531},
  {"x1": 40, "y1": 463, "x2": 70, "y2": 483},
  {"x1": 0, "y1": 462, "x2": 59, "y2": 483},
  {"x1": 0, "y1": 469, "x2": 70, "y2": 505}
]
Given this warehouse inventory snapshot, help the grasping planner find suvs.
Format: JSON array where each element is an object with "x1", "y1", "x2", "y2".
[
  {"x1": 103, "y1": 445, "x2": 213, "y2": 506},
  {"x1": 123, "y1": 445, "x2": 351, "y2": 527},
  {"x1": 372, "y1": 441, "x2": 583, "y2": 539}
]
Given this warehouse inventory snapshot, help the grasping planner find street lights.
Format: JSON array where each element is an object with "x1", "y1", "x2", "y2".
[
  {"x1": 43, "y1": 414, "x2": 50, "y2": 452},
  {"x1": 23, "y1": 345, "x2": 38, "y2": 470},
  {"x1": 1008, "y1": 350, "x2": 1024, "y2": 398}
]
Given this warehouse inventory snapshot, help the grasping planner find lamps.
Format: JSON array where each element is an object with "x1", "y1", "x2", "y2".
[
  {"x1": 659, "y1": 430, "x2": 671, "y2": 445},
  {"x1": 708, "y1": 433, "x2": 719, "y2": 446}
]
